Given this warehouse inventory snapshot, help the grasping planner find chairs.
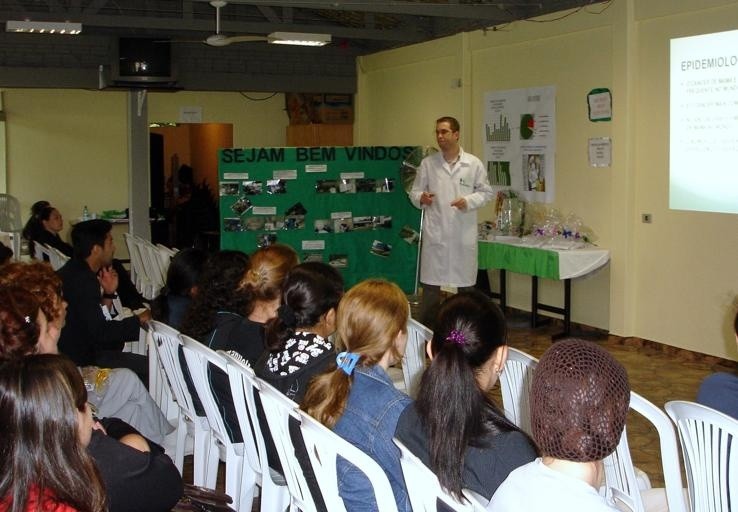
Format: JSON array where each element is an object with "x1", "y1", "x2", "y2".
[{"x1": 0, "y1": 193, "x2": 25, "y2": 262}]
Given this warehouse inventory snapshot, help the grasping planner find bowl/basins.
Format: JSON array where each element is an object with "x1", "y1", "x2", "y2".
[{"x1": 104, "y1": 210, "x2": 128, "y2": 219}]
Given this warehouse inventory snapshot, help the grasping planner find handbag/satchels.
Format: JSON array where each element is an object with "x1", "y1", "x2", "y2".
[{"x1": 171, "y1": 482, "x2": 237, "y2": 512}]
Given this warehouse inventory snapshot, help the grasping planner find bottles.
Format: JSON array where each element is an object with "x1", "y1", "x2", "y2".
[{"x1": 83, "y1": 206, "x2": 88, "y2": 221}]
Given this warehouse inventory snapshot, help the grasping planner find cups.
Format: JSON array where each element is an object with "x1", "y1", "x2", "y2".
[{"x1": 80, "y1": 364, "x2": 97, "y2": 391}]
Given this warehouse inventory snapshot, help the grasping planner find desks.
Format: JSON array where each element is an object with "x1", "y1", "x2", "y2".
[{"x1": 475, "y1": 233, "x2": 611, "y2": 343}]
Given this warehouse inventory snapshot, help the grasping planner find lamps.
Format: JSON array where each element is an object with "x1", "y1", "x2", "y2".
[
  {"x1": 267, "y1": 31, "x2": 333, "y2": 48},
  {"x1": 5, "y1": 20, "x2": 83, "y2": 36}
]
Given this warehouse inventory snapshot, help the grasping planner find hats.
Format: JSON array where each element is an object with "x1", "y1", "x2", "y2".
[{"x1": 528, "y1": 336, "x2": 631, "y2": 463}]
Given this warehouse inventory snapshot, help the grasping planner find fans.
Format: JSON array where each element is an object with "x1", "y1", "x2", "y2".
[
  {"x1": 400, "y1": 144, "x2": 442, "y2": 308},
  {"x1": 150, "y1": 1, "x2": 283, "y2": 47}
]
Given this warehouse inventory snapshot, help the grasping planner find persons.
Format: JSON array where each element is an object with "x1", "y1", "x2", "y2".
[
  {"x1": 0, "y1": 199, "x2": 631, "y2": 511},
  {"x1": 410, "y1": 115, "x2": 494, "y2": 312},
  {"x1": 694, "y1": 371, "x2": 738, "y2": 512}
]
